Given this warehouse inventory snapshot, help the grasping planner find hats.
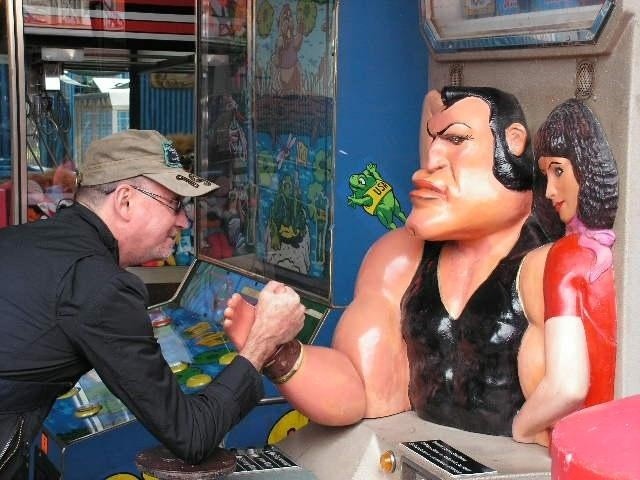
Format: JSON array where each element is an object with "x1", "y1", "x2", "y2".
[{"x1": 76, "y1": 129, "x2": 222, "y2": 197}]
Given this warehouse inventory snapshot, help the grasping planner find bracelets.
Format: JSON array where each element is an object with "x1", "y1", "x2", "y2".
[{"x1": 259, "y1": 337, "x2": 305, "y2": 385}]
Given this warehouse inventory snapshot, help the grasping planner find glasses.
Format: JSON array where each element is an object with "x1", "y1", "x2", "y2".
[{"x1": 106, "y1": 184, "x2": 184, "y2": 214}]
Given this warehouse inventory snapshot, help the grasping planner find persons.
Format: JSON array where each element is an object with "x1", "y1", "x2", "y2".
[
  {"x1": 511, "y1": 99, "x2": 618, "y2": 449},
  {"x1": 0, "y1": 129, "x2": 305, "y2": 480},
  {"x1": 216, "y1": 87, "x2": 552, "y2": 429}
]
